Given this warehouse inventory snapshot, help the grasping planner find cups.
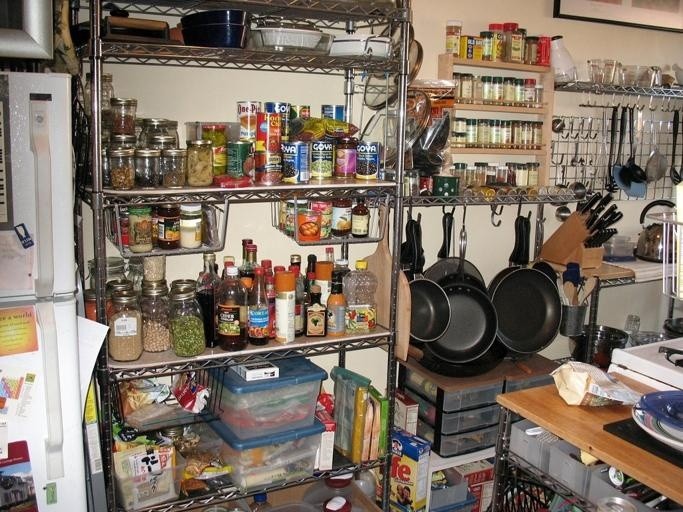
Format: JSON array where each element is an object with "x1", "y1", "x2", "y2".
[
  {"x1": 624, "y1": 314, "x2": 640, "y2": 334},
  {"x1": 432, "y1": 175, "x2": 460, "y2": 197}
]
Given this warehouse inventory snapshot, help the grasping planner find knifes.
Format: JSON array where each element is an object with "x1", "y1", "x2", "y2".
[{"x1": 578, "y1": 192, "x2": 623, "y2": 248}]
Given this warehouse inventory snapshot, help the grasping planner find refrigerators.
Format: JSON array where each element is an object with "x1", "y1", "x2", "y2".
[{"x1": 0, "y1": 69, "x2": 89, "y2": 512}]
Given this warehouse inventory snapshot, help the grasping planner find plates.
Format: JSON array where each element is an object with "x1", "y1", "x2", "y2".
[{"x1": 631, "y1": 390, "x2": 683, "y2": 452}]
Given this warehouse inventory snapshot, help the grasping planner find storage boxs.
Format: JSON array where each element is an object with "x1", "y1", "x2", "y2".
[
  {"x1": 191, "y1": 349, "x2": 328, "y2": 440},
  {"x1": 429, "y1": 459, "x2": 494, "y2": 512},
  {"x1": 397, "y1": 352, "x2": 562, "y2": 458},
  {"x1": 111, "y1": 449, "x2": 187, "y2": 512},
  {"x1": 509, "y1": 417, "x2": 683, "y2": 512},
  {"x1": 183, "y1": 474, "x2": 383, "y2": 512},
  {"x1": 190, "y1": 406, "x2": 326, "y2": 493}
]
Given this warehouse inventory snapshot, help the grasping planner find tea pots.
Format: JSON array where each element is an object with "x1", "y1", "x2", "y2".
[{"x1": 636, "y1": 200, "x2": 678, "y2": 263}]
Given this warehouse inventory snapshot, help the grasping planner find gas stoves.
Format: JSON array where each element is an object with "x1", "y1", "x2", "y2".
[{"x1": 606, "y1": 335, "x2": 683, "y2": 391}]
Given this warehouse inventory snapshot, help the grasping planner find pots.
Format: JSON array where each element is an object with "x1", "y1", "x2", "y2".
[{"x1": 405, "y1": 209, "x2": 561, "y2": 377}]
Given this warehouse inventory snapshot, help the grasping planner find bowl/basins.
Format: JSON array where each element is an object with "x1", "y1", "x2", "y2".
[
  {"x1": 180, "y1": 10, "x2": 246, "y2": 48},
  {"x1": 330, "y1": 36, "x2": 391, "y2": 58},
  {"x1": 587, "y1": 59, "x2": 654, "y2": 88},
  {"x1": 255, "y1": 28, "x2": 322, "y2": 49},
  {"x1": 568, "y1": 323, "x2": 629, "y2": 367}
]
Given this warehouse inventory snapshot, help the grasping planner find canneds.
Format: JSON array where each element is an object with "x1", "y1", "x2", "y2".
[
  {"x1": 84, "y1": 70, "x2": 382, "y2": 190},
  {"x1": 162, "y1": 425, "x2": 184, "y2": 451},
  {"x1": 444, "y1": 23, "x2": 551, "y2": 188},
  {"x1": 83, "y1": 256, "x2": 205, "y2": 362},
  {"x1": 111, "y1": 203, "x2": 208, "y2": 253},
  {"x1": 280, "y1": 198, "x2": 352, "y2": 244}
]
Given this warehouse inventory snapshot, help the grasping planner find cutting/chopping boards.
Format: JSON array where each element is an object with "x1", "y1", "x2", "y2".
[{"x1": 359, "y1": 205, "x2": 412, "y2": 362}]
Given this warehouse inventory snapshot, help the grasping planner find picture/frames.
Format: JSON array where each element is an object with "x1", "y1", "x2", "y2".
[
  {"x1": 552, "y1": 0, "x2": 683, "y2": 33},
  {"x1": 0, "y1": 0, "x2": 54, "y2": 59}
]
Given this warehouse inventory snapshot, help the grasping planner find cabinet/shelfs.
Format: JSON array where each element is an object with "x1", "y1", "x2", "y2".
[
  {"x1": 438, "y1": 52, "x2": 555, "y2": 192},
  {"x1": 491, "y1": 372, "x2": 683, "y2": 512},
  {"x1": 425, "y1": 444, "x2": 497, "y2": 512},
  {"x1": 65, "y1": 0, "x2": 410, "y2": 512},
  {"x1": 538, "y1": 255, "x2": 683, "y2": 363}
]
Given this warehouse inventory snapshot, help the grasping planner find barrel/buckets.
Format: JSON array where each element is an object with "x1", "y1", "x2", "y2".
[{"x1": 344, "y1": 259, "x2": 378, "y2": 331}]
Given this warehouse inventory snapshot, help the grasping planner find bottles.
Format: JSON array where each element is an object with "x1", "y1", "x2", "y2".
[
  {"x1": 445, "y1": 20, "x2": 541, "y2": 186},
  {"x1": 550, "y1": 35, "x2": 579, "y2": 85},
  {"x1": 84, "y1": 253, "x2": 206, "y2": 362},
  {"x1": 195, "y1": 240, "x2": 377, "y2": 351},
  {"x1": 85, "y1": 72, "x2": 187, "y2": 188},
  {"x1": 459, "y1": 183, "x2": 575, "y2": 202},
  {"x1": 248, "y1": 492, "x2": 272, "y2": 512},
  {"x1": 350, "y1": 197, "x2": 370, "y2": 238}
]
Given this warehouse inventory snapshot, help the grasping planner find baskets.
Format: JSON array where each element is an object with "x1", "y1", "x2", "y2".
[{"x1": 464, "y1": 468, "x2": 494, "y2": 512}]
